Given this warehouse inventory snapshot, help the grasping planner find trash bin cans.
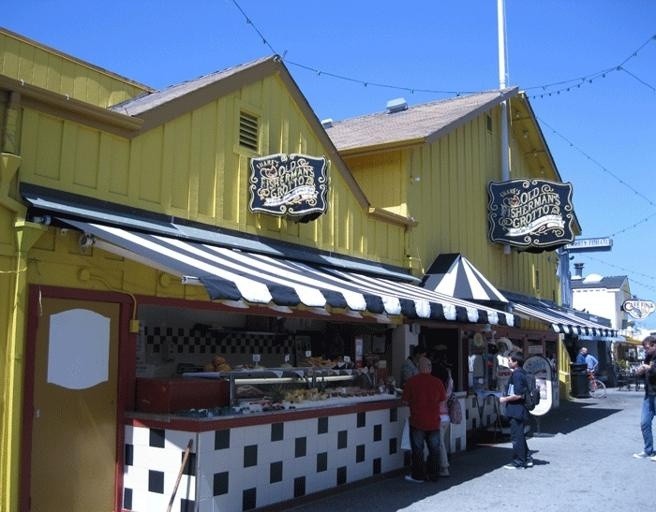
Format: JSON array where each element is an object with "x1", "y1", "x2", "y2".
[{"x1": 570, "y1": 363, "x2": 588, "y2": 398}]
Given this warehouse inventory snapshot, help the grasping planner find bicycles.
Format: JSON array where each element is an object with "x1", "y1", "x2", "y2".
[{"x1": 587, "y1": 368, "x2": 608, "y2": 400}]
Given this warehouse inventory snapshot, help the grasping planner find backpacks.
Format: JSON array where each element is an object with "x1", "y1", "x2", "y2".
[{"x1": 511, "y1": 369, "x2": 541, "y2": 412}]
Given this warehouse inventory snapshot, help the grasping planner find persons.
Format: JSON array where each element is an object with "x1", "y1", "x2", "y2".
[
  {"x1": 584, "y1": 353, "x2": 599, "y2": 392},
  {"x1": 401, "y1": 357, "x2": 447, "y2": 484},
  {"x1": 575, "y1": 346, "x2": 588, "y2": 365},
  {"x1": 498, "y1": 351, "x2": 534, "y2": 471},
  {"x1": 428, "y1": 357, "x2": 455, "y2": 477},
  {"x1": 400, "y1": 344, "x2": 428, "y2": 388},
  {"x1": 632, "y1": 334, "x2": 656, "y2": 462}
]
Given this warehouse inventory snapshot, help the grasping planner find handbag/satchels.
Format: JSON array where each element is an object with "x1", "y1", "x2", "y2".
[{"x1": 447, "y1": 393, "x2": 463, "y2": 425}]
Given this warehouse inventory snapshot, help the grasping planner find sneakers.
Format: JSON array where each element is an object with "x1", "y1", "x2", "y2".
[
  {"x1": 404, "y1": 463, "x2": 451, "y2": 484},
  {"x1": 504, "y1": 460, "x2": 535, "y2": 470},
  {"x1": 634, "y1": 449, "x2": 656, "y2": 460}
]
